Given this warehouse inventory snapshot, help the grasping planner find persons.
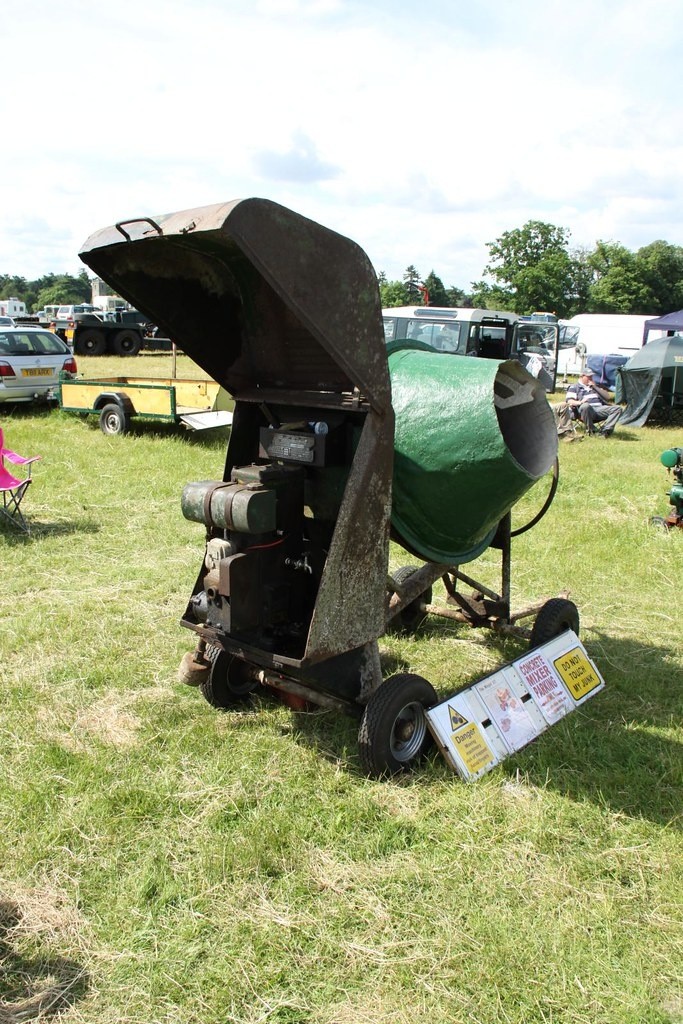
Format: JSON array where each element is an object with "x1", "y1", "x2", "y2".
[{"x1": 566, "y1": 368, "x2": 622, "y2": 438}]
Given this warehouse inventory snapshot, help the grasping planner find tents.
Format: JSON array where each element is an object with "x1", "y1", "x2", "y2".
[
  {"x1": 642, "y1": 310, "x2": 683, "y2": 346},
  {"x1": 615, "y1": 336, "x2": 683, "y2": 428}
]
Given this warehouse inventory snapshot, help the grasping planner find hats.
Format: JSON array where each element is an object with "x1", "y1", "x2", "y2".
[{"x1": 580, "y1": 368, "x2": 596, "y2": 377}]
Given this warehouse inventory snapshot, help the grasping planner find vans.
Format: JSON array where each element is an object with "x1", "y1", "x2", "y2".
[
  {"x1": 539, "y1": 314, "x2": 683, "y2": 376},
  {"x1": 379, "y1": 306, "x2": 560, "y2": 394}
]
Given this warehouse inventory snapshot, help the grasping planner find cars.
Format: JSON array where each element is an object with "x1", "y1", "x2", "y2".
[{"x1": 1, "y1": 316, "x2": 20, "y2": 343}]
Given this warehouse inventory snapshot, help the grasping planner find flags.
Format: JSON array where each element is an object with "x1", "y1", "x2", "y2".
[{"x1": 424, "y1": 291, "x2": 428, "y2": 304}]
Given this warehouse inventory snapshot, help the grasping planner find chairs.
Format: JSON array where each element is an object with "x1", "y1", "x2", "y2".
[
  {"x1": 416, "y1": 334, "x2": 436, "y2": 347},
  {"x1": 16, "y1": 343, "x2": 29, "y2": 351},
  {"x1": 0, "y1": 425, "x2": 43, "y2": 536},
  {"x1": 565, "y1": 384, "x2": 607, "y2": 435}
]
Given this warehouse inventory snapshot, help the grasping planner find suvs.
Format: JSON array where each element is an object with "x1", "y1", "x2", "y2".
[
  {"x1": 56, "y1": 305, "x2": 102, "y2": 323},
  {"x1": 0, "y1": 324, "x2": 77, "y2": 407}
]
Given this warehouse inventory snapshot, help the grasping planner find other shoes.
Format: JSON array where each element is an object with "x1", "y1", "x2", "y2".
[{"x1": 601, "y1": 431, "x2": 608, "y2": 436}]
